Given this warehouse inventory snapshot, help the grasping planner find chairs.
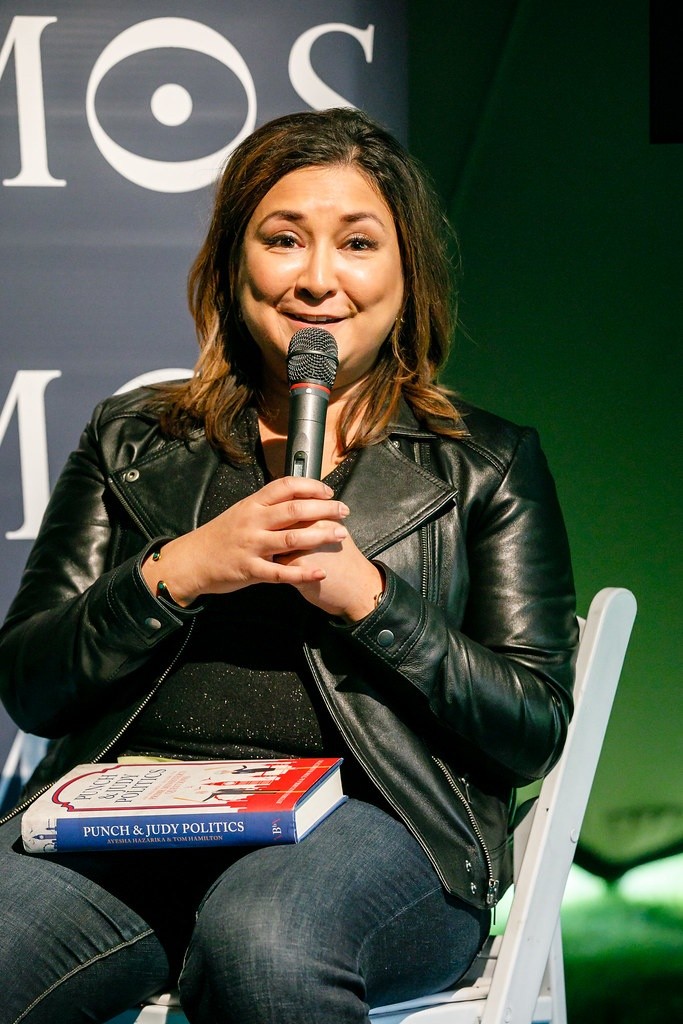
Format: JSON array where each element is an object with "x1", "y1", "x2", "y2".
[{"x1": 103, "y1": 587, "x2": 637, "y2": 1024}]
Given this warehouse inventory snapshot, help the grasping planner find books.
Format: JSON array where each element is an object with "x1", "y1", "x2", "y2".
[{"x1": 21, "y1": 757, "x2": 349, "y2": 854}]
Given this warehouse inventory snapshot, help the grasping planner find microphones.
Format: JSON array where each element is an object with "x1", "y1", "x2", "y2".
[{"x1": 282, "y1": 327, "x2": 338, "y2": 482}]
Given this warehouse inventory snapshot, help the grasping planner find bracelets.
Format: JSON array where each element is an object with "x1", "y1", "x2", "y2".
[
  {"x1": 151, "y1": 548, "x2": 184, "y2": 610},
  {"x1": 373, "y1": 590, "x2": 383, "y2": 608}
]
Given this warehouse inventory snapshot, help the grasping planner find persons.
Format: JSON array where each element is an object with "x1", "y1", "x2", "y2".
[{"x1": 0, "y1": 106, "x2": 581, "y2": 1024}]
